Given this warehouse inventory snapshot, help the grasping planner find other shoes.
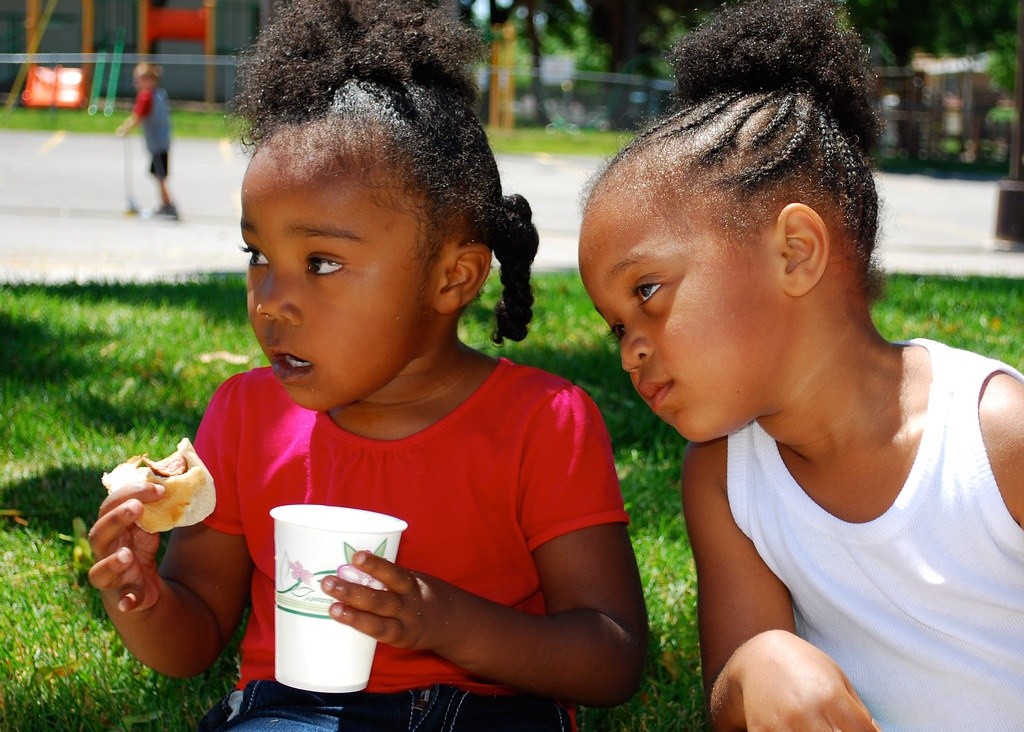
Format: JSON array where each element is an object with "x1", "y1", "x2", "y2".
[{"x1": 151, "y1": 205, "x2": 179, "y2": 221}]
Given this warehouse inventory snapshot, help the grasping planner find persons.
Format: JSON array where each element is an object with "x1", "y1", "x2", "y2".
[
  {"x1": 115, "y1": 61, "x2": 180, "y2": 224},
  {"x1": 88, "y1": 4, "x2": 652, "y2": 730},
  {"x1": 578, "y1": 3, "x2": 964, "y2": 730}
]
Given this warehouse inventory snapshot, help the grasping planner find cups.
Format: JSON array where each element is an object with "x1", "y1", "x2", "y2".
[{"x1": 270, "y1": 504, "x2": 408, "y2": 693}]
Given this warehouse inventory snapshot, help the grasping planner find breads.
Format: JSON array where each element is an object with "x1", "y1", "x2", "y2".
[{"x1": 102, "y1": 436, "x2": 217, "y2": 534}]
